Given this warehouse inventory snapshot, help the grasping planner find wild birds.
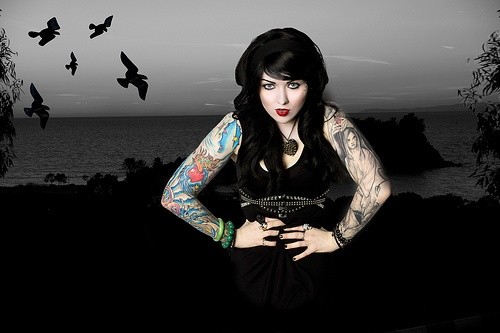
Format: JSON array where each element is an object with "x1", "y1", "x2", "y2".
[
  {"x1": 28, "y1": 17, "x2": 60, "y2": 46},
  {"x1": 117, "y1": 50, "x2": 148, "y2": 101},
  {"x1": 65, "y1": 52, "x2": 79, "y2": 76},
  {"x1": 23, "y1": 82, "x2": 50, "y2": 131},
  {"x1": 89, "y1": 14, "x2": 114, "y2": 39}
]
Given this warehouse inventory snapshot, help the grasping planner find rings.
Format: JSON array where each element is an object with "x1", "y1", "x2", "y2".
[
  {"x1": 302, "y1": 232, "x2": 305, "y2": 240},
  {"x1": 262, "y1": 239, "x2": 265, "y2": 246},
  {"x1": 302, "y1": 224, "x2": 312, "y2": 230},
  {"x1": 255, "y1": 215, "x2": 265, "y2": 224},
  {"x1": 261, "y1": 222, "x2": 267, "y2": 231}
]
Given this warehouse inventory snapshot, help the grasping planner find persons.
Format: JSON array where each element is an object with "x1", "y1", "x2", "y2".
[{"x1": 161, "y1": 27, "x2": 392, "y2": 311}]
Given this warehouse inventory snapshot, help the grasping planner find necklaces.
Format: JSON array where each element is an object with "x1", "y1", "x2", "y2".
[{"x1": 276, "y1": 117, "x2": 298, "y2": 156}]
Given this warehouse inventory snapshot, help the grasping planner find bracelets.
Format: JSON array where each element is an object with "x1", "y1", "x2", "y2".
[
  {"x1": 213, "y1": 218, "x2": 236, "y2": 249},
  {"x1": 332, "y1": 223, "x2": 349, "y2": 250}
]
